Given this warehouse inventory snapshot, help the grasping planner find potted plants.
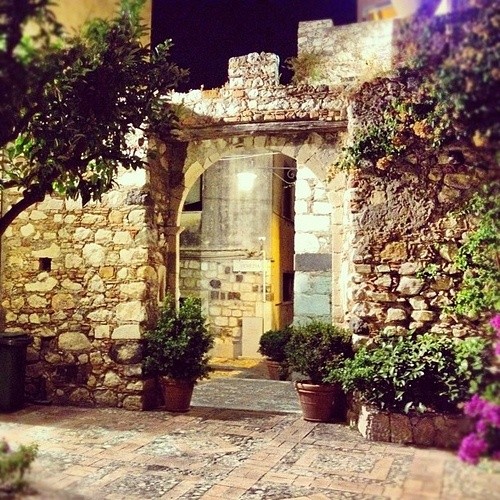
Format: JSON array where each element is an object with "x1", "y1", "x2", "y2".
[
  {"x1": 285, "y1": 316, "x2": 356, "y2": 421},
  {"x1": 139, "y1": 286, "x2": 215, "y2": 412},
  {"x1": 257, "y1": 330, "x2": 294, "y2": 382}
]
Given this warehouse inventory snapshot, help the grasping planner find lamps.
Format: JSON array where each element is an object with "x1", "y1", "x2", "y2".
[{"x1": 232, "y1": 166, "x2": 297, "y2": 190}]
